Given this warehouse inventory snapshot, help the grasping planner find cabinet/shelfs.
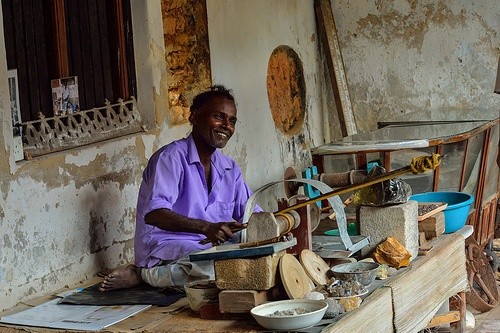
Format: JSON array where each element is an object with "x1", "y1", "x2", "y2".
[{"x1": 309, "y1": 117, "x2": 500, "y2": 250}]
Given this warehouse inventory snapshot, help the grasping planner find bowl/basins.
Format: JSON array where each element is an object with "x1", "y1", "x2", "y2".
[
  {"x1": 331, "y1": 262, "x2": 380, "y2": 288},
  {"x1": 184, "y1": 280, "x2": 219, "y2": 313},
  {"x1": 410, "y1": 191, "x2": 475, "y2": 234},
  {"x1": 250, "y1": 299, "x2": 329, "y2": 331},
  {"x1": 312, "y1": 284, "x2": 369, "y2": 312}
]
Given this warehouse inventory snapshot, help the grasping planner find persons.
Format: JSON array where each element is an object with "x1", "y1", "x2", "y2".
[
  {"x1": 98, "y1": 85, "x2": 264, "y2": 293},
  {"x1": 61, "y1": 82, "x2": 70, "y2": 115}
]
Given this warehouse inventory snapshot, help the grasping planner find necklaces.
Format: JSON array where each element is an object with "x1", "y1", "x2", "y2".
[{"x1": 206, "y1": 178, "x2": 211, "y2": 185}]
